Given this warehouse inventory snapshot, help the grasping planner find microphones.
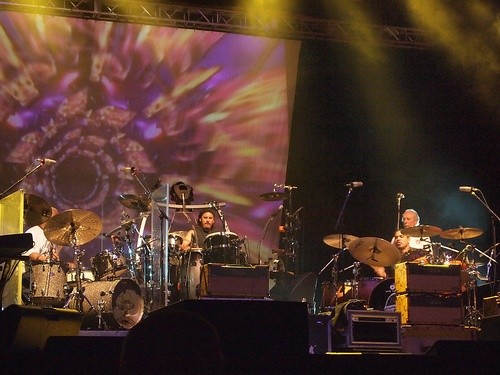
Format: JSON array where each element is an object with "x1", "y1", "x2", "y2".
[
  {"x1": 397, "y1": 193, "x2": 405, "y2": 198},
  {"x1": 120, "y1": 167, "x2": 139, "y2": 172},
  {"x1": 461, "y1": 245, "x2": 473, "y2": 254},
  {"x1": 36, "y1": 159, "x2": 56, "y2": 164},
  {"x1": 345, "y1": 182, "x2": 363, "y2": 188},
  {"x1": 270, "y1": 205, "x2": 283, "y2": 221},
  {"x1": 459, "y1": 186, "x2": 479, "y2": 192}
]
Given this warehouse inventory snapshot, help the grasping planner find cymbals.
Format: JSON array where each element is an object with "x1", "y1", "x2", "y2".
[
  {"x1": 258, "y1": 192, "x2": 291, "y2": 201},
  {"x1": 349, "y1": 238, "x2": 401, "y2": 266},
  {"x1": 118, "y1": 195, "x2": 157, "y2": 213},
  {"x1": 137, "y1": 238, "x2": 157, "y2": 248},
  {"x1": 440, "y1": 229, "x2": 484, "y2": 239},
  {"x1": 106, "y1": 214, "x2": 146, "y2": 235},
  {"x1": 400, "y1": 226, "x2": 441, "y2": 237},
  {"x1": 43, "y1": 209, "x2": 102, "y2": 246},
  {"x1": 323, "y1": 234, "x2": 358, "y2": 250}
]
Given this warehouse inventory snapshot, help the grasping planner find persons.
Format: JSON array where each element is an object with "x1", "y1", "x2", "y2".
[
  {"x1": 21, "y1": 206, "x2": 75, "y2": 269},
  {"x1": 387, "y1": 210, "x2": 434, "y2": 274},
  {"x1": 180, "y1": 211, "x2": 221, "y2": 298}
]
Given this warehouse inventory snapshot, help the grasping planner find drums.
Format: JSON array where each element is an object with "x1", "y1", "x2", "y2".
[
  {"x1": 93, "y1": 248, "x2": 128, "y2": 279},
  {"x1": 29, "y1": 261, "x2": 69, "y2": 303},
  {"x1": 65, "y1": 269, "x2": 95, "y2": 284},
  {"x1": 71, "y1": 278, "x2": 144, "y2": 330},
  {"x1": 359, "y1": 276, "x2": 382, "y2": 304},
  {"x1": 204, "y1": 231, "x2": 240, "y2": 252},
  {"x1": 143, "y1": 234, "x2": 183, "y2": 252},
  {"x1": 367, "y1": 277, "x2": 396, "y2": 313},
  {"x1": 180, "y1": 248, "x2": 205, "y2": 301},
  {"x1": 321, "y1": 281, "x2": 359, "y2": 313}
]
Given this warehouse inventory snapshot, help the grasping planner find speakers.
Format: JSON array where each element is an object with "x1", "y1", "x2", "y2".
[
  {"x1": 0, "y1": 298, "x2": 312, "y2": 375},
  {"x1": 209, "y1": 263, "x2": 270, "y2": 299},
  {"x1": 394, "y1": 262, "x2": 464, "y2": 327},
  {"x1": 269, "y1": 271, "x2": 318, "y2": 301}
]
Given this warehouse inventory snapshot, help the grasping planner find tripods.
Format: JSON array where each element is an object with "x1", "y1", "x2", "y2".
[{"x1": 62, "y1": 226, "x2": 109, "y2": 330}]
